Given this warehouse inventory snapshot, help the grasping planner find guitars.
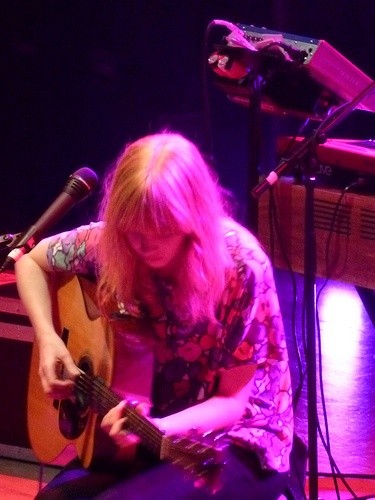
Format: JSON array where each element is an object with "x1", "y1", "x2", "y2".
[{"x1": 25, "y1": 266, "x2": 228, "y2": 496}]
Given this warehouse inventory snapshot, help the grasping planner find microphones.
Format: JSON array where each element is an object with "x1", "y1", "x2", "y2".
[{"x1": 2, "y1": 167, "x2": 99, "y2": 270}]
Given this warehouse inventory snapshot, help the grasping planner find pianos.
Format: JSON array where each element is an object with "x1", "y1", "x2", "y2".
[{"x1": 205, "y1": 19, "x2": 374, "y2": 123}]
